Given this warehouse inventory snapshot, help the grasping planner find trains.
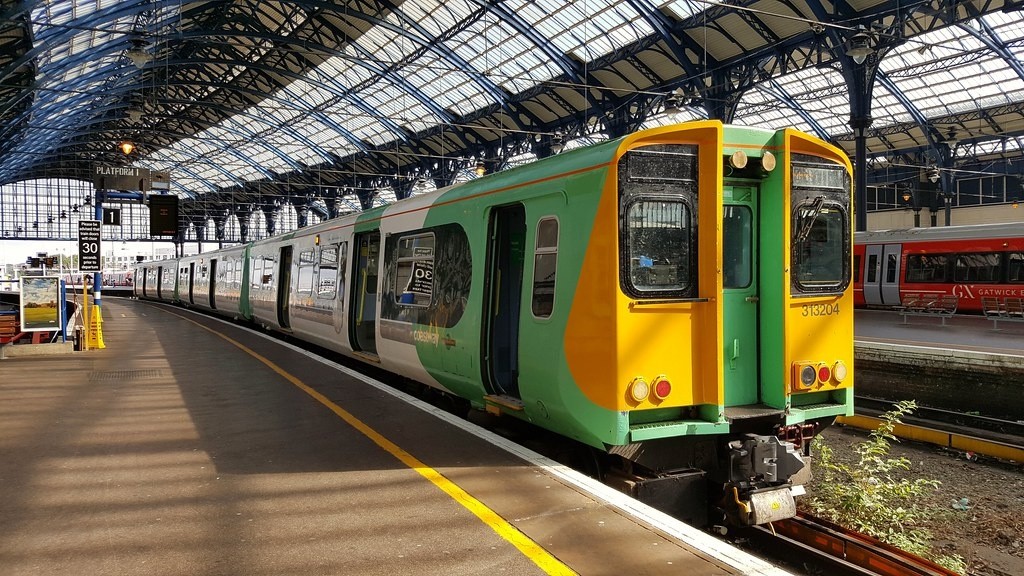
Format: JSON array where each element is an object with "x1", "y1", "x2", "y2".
[
  {"x1": 132, "y1": 114, "x2": 854, "y2": 530},
  {"x1": 854, "y1": 222, "x2": 1024, "y2": 317}
]
